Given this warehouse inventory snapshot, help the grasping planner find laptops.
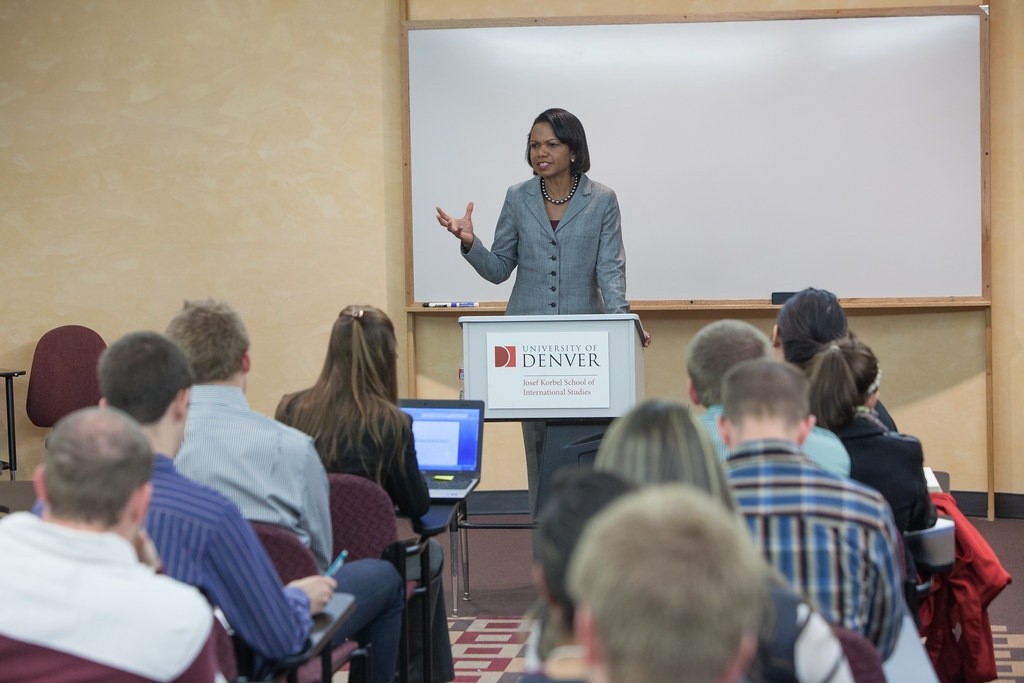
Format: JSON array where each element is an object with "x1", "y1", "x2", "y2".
[{"x1": 397, "y1": 399, "x2": 485, "y2": 499}]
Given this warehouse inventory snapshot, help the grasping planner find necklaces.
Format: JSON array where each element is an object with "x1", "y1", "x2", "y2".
[{"x1": 540, "y1": 171, "x2": 578, "y2": 203}]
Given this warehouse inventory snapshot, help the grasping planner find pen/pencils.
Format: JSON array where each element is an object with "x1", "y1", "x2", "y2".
[{"x1": 326, "y1": 548, "x2": 349, "y2": 580}]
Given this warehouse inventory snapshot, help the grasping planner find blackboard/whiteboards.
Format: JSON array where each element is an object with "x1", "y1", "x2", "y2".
[{"x1": 395, "y1": 4, "x2": 991, "y2": 306}]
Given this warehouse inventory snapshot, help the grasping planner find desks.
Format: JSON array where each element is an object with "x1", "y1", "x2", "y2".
[{"x1": 394, "y1": 498, "x2": 460, "y2": 536}]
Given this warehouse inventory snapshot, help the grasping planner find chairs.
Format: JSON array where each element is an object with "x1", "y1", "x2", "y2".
[
  {"x1": 27, "y1": 325, "x2": 108, "y2": 450},
  {"x1": 449, "y1": 489, "x2": 534, "y2": 618},
  {"x1": 249, "y1": 522, "x2": 370, "y2": 683},
  {"x1": 326, "y1": 473, "x2": 432, "y2": 683}
]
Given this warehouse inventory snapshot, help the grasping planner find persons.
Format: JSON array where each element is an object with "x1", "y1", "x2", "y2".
[
  {"x1": 0, "y1": 286, "x2": 936, "y2": 683},
  {"x1": 434, "y1": 108, "x2": 651, "y2": 532}
]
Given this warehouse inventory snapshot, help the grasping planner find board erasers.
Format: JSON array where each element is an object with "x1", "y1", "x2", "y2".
[{"x1": 771, "y1": 291, "x2": 798, "y2": 304}]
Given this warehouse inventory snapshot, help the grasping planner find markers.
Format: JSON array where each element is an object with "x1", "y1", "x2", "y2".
[{"x1": 423, "y1": 301, "x2": 480, "y2": 308}]
